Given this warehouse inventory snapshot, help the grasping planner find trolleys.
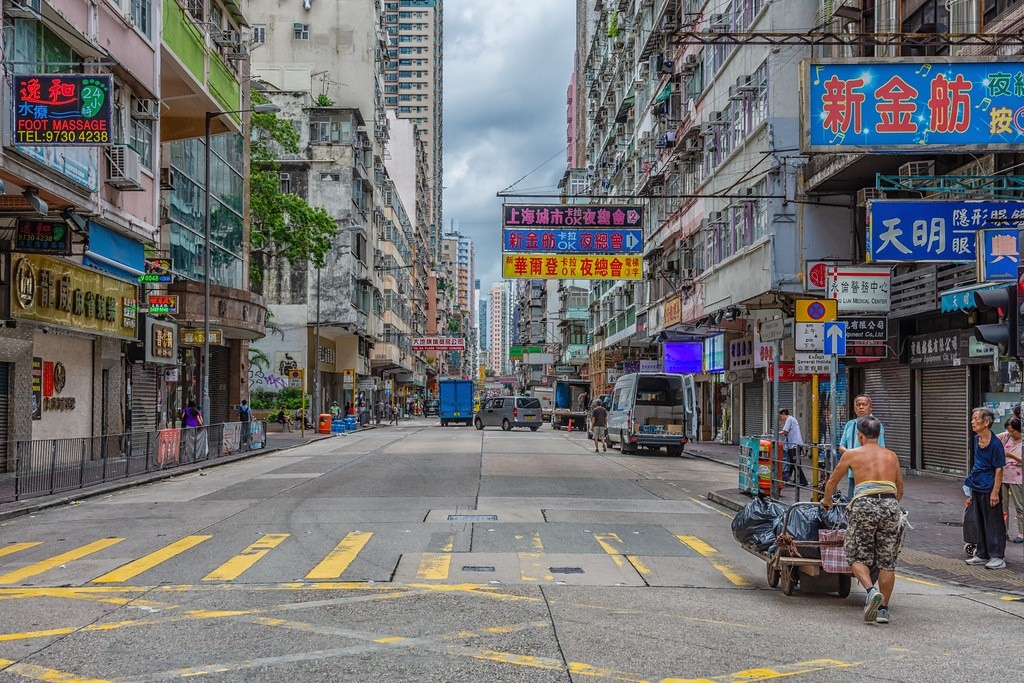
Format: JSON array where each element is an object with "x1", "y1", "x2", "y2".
[{"x1": 766, "y1": 501, "x2": 908, "y2": 599}]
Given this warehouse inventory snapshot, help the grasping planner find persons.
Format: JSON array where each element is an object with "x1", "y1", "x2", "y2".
[
  {"x1": 348, "y1": 403, "x2": 354, "y2": 414},
  {"x1": 278, "y1": 406, "x2": 293, "y2": 432},
  {"x1": 839, "y1": 394, "x2": 885, "y2": 498},
  {"x1": 772, "y1": 408, "x2": 808, "y2": 486},
  {"x1": 592, "y1": 399, "x2": 608, "y2": 452},
  {"x1": 996, "y1": 416, "x2": 1024, "y2": 543},
  {"x1": 374, "y1": 400, "x2": 391, "y2": 417},
  {"x1": 182, "y1": 399, "x2": 202, "y2": 428},
  {"x1": 689, "y1": 400, "x2": 701, "y2": 444},
  {"x1": 331, "y1": 401, "x2": 341, "y2": 419},
  {"x1": 820, "y1": 415, "x2": 904, "y2": 622},
  {"x1": 296, "y1": 408, "x2": 310, "y2": 430},
  {"x1": 1013, "y1": 406, "x2": 1021, "y2": 419},
  {"x1": 390, "y1": 404, "x2": 399, "y2": 425},
  {"x1": 238, "y1": 400, "x2": 252, "y2": 442},
  {"x1": 345, "y1": 402, "x2": 350, "y2": 417},
  {"x1": 965, "y1": 408, "x2": 1007, "y2": 569},
  {"x1": 357, "y1": 399, "x2": 362, "y2": 422},
  {"x1": 578, "y1": 392, "x2": 587, "y2": 412}
]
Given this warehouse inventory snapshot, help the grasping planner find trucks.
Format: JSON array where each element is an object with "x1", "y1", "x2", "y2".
[
  {"x1": 551, "y1": 379, "x2": 592, "y2": 430},
  {"x1": 531, "y1": 386, "x2": 554, "y2": 423},
  {"x1": 438, "y1": 380, "x2": 474, "y2": 426}
]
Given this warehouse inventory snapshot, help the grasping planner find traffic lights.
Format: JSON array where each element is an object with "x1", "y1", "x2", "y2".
[
  {"x1": 1017, "y1": 265, "x2": 1024, "y2": 357},
  {"x1": 974, "y1": 284, "x2": 1015, "y2": 357}
]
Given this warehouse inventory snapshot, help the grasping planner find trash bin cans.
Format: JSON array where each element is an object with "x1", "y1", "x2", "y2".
[
  {"x1": 319, "y1": 413, "x2": 332, "y2": 433},
  {"x1": 360, "y1": 411, "x2": 369, "y2": 426},
  {"x1": 757, "y1": 438, "x2": 783, "y2": 496}
]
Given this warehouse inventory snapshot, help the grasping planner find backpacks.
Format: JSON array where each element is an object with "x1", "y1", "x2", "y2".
[{"x1": 240, "y1": 407, "x2": 249, "y2": 421}]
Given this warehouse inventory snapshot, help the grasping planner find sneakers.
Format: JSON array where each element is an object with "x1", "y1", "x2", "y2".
[
  {"x1": 864, "y1": 589, "x2": 884, "y2": 621},
  {"x1": 965, "y1": 557, "x2": 989, "y2": 565},
  {"x1": 985, "y1": 558, "x2": 1007, "y2": 568},
  {"x1": 876, "y1": 609, "x2": 891, "y2": 623}
]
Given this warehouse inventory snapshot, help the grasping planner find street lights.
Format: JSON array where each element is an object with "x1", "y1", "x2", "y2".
[
  {"x1": 313, "y1": 225, "x2": 364, "y2": 435},
  {"x1": 201, "y1": 103, "x2": 280, "y2": 454}
]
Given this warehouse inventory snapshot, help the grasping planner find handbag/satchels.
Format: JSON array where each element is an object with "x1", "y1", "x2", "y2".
[{"x1": 819, "y1": 512, "x2": 853, "y2": 574}]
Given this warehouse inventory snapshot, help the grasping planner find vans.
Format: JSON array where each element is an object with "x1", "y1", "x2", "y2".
[
  {"x1": 422, "y1": 399, "x2": 439, "y2": 417},
  {"x1": 587, "y1": 395, "x2": 612, "y2": 439},
  {"x1": 605, "y1": 373, "x2": 698, "y2": 457},
  {"x1": 473, "y1": 396, "x2": 543, "y2": 431}
]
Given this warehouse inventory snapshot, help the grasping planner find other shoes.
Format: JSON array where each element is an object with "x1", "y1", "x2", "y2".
[
  {"x1": 603, "y1": 445, "x2": 606, "y2": 452},
  {"x1": 1014, "y1": 536, "x2": 1024, "y2": 542},
  {"x1": 802, "y1": 482, "x2": 809, "y2": 486},
  {"x1": 595, "y1": 450, "x2": 599, "y2": 452}
]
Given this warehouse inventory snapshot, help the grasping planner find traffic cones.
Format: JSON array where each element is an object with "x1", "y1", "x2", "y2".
[{"x1": 567, "y1": 419, "x2": 572, "y2": 432}]
